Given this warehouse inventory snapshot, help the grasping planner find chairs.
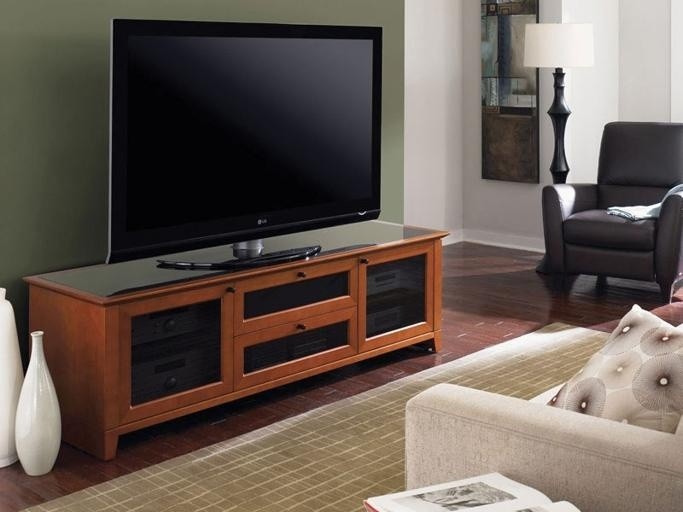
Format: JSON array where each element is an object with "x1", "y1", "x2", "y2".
[
  {"x1": 407, "y1": 370, "x2": 682, "y2": 511},
  {"x1": 541, "y1": 120, "x2": 682, "y2": 303}
]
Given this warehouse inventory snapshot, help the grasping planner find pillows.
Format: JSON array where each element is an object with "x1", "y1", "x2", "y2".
[{"x1": 546, "y1": 303, "x2": 683, "y2": 437}]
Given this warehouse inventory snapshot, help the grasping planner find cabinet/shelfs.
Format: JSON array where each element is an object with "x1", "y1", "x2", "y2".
[{"x1": 23, "y1": 220, "x2": 451, "y2": 463}]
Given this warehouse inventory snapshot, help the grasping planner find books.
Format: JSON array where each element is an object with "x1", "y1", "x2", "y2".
[{"x1": 362, "y1": 469, "x2": 582, "y2": 512}]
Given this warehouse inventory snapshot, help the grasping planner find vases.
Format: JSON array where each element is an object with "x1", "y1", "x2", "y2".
[{"x1": 0, "y1": 287, "x2": 63, "y2": 478}]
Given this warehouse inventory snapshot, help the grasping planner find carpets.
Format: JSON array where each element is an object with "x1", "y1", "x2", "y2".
[{"x1": 15, "y1": 321, "x2": 615, "y2": 510}]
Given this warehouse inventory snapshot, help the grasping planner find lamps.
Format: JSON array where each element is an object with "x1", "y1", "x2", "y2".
[{"x1": 522, "y1": 23, "x2": 596, "y2": 277}]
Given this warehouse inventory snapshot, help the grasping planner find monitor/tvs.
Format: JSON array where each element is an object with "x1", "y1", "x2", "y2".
[{"x1": 103, "y1": 17, "x2": 383, "y2": 273}]
